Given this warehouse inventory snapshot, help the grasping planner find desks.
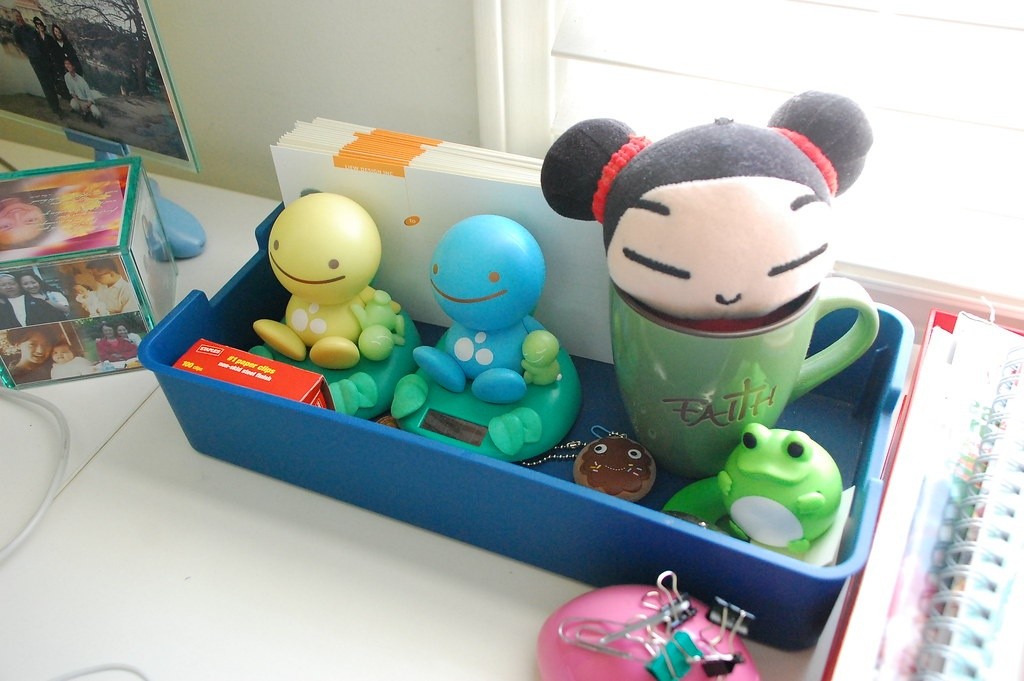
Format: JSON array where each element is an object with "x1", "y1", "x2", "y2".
[{"x1": 0, "y1": 136, "x2": 850, "y2": 681}]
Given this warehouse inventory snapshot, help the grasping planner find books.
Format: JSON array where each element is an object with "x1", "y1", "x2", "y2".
[{"x1": 820, "y1": 307, "x2": 1024, "y2": 681}]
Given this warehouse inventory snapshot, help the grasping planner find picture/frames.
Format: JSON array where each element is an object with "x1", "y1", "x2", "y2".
[{"x1": 0, "y1": 0, "x2": 203, "y2": 176}]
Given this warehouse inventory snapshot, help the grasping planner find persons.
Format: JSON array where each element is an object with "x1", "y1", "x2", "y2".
[
  {"x1": 6, "y1": 8, "x2": 107, "y2": 130},
  {"x1": 0, "y1": 189, "x2": 175, "y2": 388}
]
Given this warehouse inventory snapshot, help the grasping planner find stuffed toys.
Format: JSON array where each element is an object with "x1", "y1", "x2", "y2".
[{"x1": 540, "y1": 90, "x2": 873, "y2": 334}]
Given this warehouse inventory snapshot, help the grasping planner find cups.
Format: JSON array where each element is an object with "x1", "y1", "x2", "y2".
[{"x1": 607, "y1": 273, "x2": 880, "y2": 476}]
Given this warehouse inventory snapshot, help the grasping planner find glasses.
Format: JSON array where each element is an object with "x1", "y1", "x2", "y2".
[{"x1": 35, "y1": 21, "x2": 43, "y2": 26}]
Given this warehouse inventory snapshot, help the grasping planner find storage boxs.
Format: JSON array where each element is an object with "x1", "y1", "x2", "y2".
[
  {"x1": 138, "y1": 202, "x2": 915, "y2": 657},
  {"x1": 173, "y1": 336, "x2": 336, "y2": 414},
  {"x1": 0, "y1": 156, "x2": 179, "y2": 391}
]
